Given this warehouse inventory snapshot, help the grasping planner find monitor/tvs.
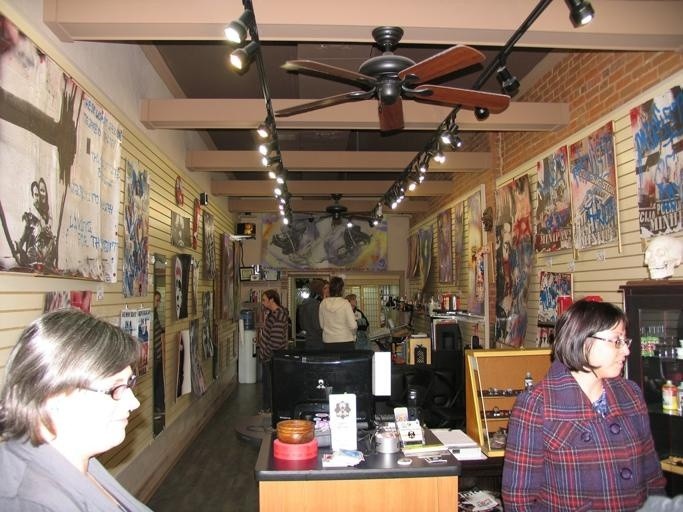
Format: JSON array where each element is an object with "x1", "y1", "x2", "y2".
[{"x1": 271, "y1": 351, "x2": 375, "y2": 431}]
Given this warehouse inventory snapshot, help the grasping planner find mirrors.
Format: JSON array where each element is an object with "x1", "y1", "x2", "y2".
[{"x1": 153, "y1": 252, "x2": 166, "y2": 438}]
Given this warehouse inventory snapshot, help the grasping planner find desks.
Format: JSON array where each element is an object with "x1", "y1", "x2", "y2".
[{"x1": 253, "y1": 426, "x2": 462, "y2": 512}]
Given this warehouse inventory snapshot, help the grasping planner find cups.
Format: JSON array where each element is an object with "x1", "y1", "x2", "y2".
[
  {"x1": 374, "y1": 431, "x2": 399, "y2": 454},
  {"x1": 639, "y1": 324, "x2": 682, "y2": 359}
]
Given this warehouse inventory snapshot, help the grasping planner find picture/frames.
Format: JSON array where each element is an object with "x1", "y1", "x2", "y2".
[
  {"x1": 239, "y1": 267, "x2": 255, "y2": 281},
  {"x1": 220, "y1": 232, "x2": 243, "y2": 322},
  {"x1": 265, "y1": 271, "x2": 278, "y2": 281}
]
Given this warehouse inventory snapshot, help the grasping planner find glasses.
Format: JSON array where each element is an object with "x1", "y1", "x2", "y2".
[
  {"x1": 588, "y1": 334, "x2": 634, "y2": 351},
  {"x1": 76, "y1": 373, "x2": 138, "y2": 401}
]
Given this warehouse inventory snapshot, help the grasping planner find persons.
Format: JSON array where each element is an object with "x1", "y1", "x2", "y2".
[
  {"x1": 297, "y1": 277, "x2": 370, "y2": 352},
  {"x1": 0, "y1": 304, "x2": 154, "y2": 512},
  {"x1": 256, "y1": 289, "x2": 289, "y2": 416},
  {"x1": 153, "y1": 290, "x2": 165, "y2": 421},
  {"x1": 496, "y1": 222, "x2": 516, "y2": 318},
  {"x1": 15, "y1": 177, "x2": 53, "y2": 261},
  {"x1": 175, "y1": 258, "x2": 183, "y2": 319},
  {"x1": 653, "y1": 177, "x2": 677, "y2": 212},
  {"x1": 500, "y1": 299, "x2": 668, "y2": 512}
]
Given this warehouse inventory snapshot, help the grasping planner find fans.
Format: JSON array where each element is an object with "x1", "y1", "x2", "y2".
[
  {"x1": 302, "y1": 193, "x2": 384, "y2": 222},
  {"x1": 273, "y1": 26, "x2": 511, "y2": 131}
]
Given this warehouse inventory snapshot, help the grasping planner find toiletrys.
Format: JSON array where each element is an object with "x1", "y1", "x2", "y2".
[{"x1": 442, "y1": 294, "x2": 461, "y2": 310}]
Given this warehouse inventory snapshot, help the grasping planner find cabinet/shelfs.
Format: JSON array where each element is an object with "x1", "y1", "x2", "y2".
[{"x1": 620, "y1": 285, "x2": 683, "y2": 499}]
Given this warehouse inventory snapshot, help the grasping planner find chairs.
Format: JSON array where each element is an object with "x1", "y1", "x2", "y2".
[{"x1": 386, "y1": 344, "x2": 483, "y2": 431}]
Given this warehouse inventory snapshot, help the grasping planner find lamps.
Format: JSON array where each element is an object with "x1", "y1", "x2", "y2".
[
  {"x1": 224, "y1": 0, "x2": 293, "y2": 231},
  {"x1": 369, "y1": 0, "x2": 594, "y2": 226}
]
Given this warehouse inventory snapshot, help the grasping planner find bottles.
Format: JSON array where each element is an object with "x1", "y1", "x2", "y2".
[
  {"x1": 523, "y1": 371, "x2": 534, "y2": 392},
  {"x1": 406, "y1": 388, "x2": 419, "y2": 426},
  {"x1": 660, "y1": 378, "x2": 682, "y2": 414},
  {"x1": 392, "y1": 294, "x2": 456, "y2": 318}
]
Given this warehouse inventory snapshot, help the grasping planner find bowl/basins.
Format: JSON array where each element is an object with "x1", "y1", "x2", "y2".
[{"x1": 275, "y1": 418, "x2": 315, "y2": 444}]
